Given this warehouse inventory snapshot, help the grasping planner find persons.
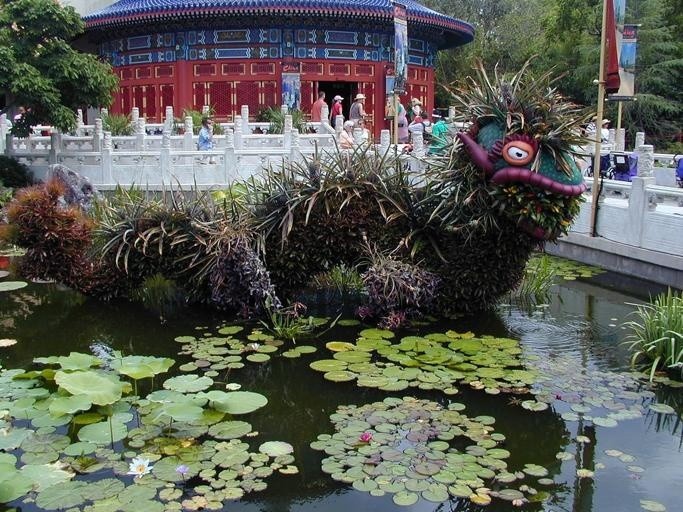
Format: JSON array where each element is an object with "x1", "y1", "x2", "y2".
[
  {"x1": 311, "y1": 91, "x2": 371, "y2": 150},
  {"x1": 601, "y1": 119, "x2": 611, "y2": 144},
  {"x1": 197, "y1": 117, "x2": 217, "y2": 164},
  {"x1": 585, "y1": 115, "x2": 597, "y2": 135},
  {"x1": 385, "y1": 96, "x2": 449, "y2": 158},
  {"x1": 13, "y1": 107, "x2": 26, "y2": 123}
]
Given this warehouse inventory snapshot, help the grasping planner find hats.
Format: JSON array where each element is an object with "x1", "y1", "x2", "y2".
[
  {"x1": 415, "y1": 116, "x2": 423, "y2": 123},
  {"x1": 353, "y1": 94, "x2": 367, "y2": 101},
  {"x1": 334, "y1": 95, "x2": 344, "y2": 100}
]
